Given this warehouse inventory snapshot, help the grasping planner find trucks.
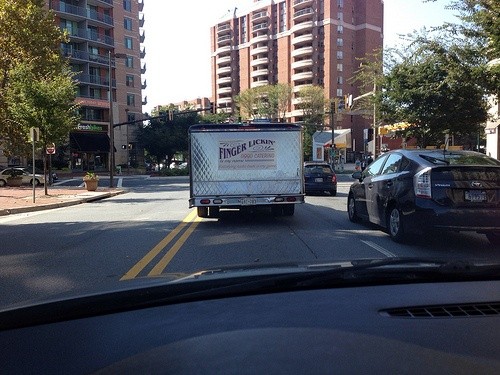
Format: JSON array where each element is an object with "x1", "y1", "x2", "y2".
[{"x1": 188, "y1": 122, "x2": 305, "y2": 216}]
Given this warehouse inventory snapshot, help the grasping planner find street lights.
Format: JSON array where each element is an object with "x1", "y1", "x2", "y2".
[{"x1": 109, "y1": 51, "x2": 128, "y2": 189}]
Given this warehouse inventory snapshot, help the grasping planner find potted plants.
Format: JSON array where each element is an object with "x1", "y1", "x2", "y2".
[{"x1": 83, "y1": 172, "x2": 99, "y2": 191}]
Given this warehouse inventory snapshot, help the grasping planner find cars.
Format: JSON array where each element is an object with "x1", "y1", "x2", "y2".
[
  {"x1": 347, "y1": 149, "x2": 500, "y2": 242},
  {"x1": 304, "y1": 161, "x2": 337, "y2": 196},
  {"x1": 0, "y1": 166, "x2": 57, "y2": 187}
]
